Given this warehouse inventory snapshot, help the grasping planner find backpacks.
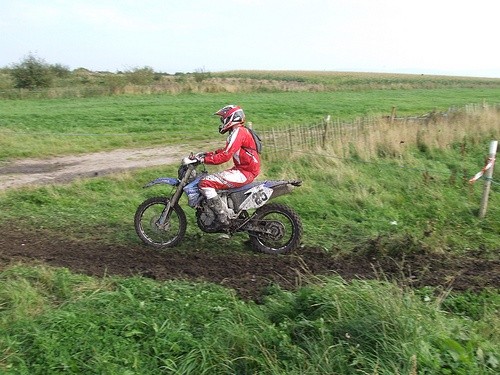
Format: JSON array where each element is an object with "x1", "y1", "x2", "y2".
[{"x1": 237, "y1": 125, "x2": 262, "y2": 154}]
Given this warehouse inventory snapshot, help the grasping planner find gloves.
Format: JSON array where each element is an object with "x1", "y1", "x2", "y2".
[{"x1": 188, "y1": 156, "x2": 204, "y2": 166}]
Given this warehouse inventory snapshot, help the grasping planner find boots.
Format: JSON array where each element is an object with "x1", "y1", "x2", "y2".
[{"x1": 203, "y1": 195, "x2": 229, "y2": 232}]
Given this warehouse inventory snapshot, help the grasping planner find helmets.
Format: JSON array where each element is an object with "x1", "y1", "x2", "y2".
[{"x1": 214, "y1": 105, "x2": 246, "y2": 134}]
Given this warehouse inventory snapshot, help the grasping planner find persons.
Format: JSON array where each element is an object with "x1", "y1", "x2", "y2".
[{"x1": 192, "y1": 104, "x2": 261, "y2": 233}]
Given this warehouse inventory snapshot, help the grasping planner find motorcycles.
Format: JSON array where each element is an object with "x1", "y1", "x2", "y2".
[{"x1": 132, "y1": 152, "x2": 304, "y2": 256}]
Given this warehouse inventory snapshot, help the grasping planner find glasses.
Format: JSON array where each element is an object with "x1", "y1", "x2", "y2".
[{"x1": 220, "y1": 117, "x2": 224, "y2": 124}]
